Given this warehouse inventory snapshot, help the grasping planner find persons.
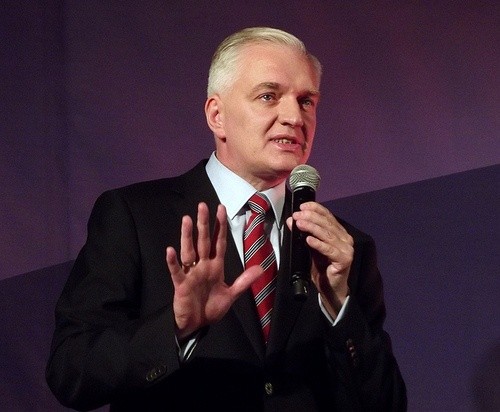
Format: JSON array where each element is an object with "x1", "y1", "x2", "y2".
[{"x1": 46, "y1": 25, "x2": 410, "y2": 411}]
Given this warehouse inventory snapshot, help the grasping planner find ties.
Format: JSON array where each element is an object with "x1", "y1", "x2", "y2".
[{"x1": 240, "y1": 192, "x2": 277, "y2": 350}]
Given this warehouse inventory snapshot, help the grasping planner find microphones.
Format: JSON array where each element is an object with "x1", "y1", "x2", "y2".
[{"x1": 290, "y1": 163, "x2": 320, "y2": 300}]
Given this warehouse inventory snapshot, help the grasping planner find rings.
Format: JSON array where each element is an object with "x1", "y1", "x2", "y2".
[{"x1": 180, "y1": 262, "x2": 198, "y2": 268}]
